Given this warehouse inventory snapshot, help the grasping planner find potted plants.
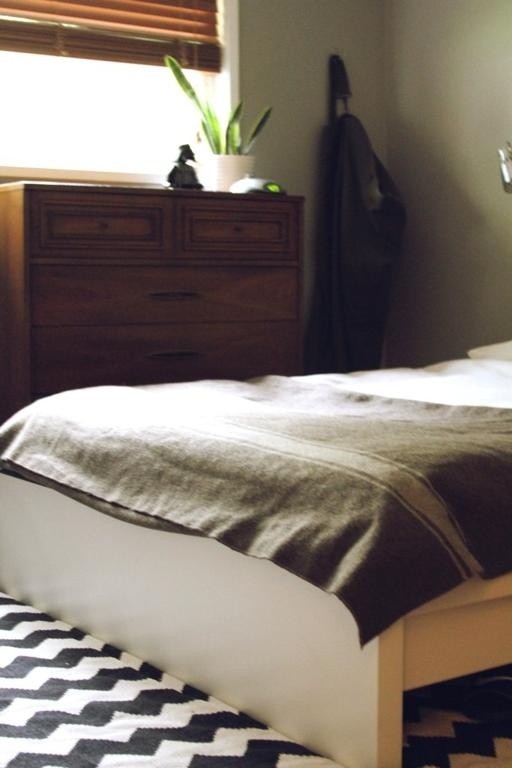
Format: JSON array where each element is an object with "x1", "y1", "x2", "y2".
[{"x1": 161, "y1": 52, "x2": 273, "y2": 192}]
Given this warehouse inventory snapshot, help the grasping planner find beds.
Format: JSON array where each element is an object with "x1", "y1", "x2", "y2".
[{"x1": 1, "y1": 339, "x2": 511, "y2": 768}]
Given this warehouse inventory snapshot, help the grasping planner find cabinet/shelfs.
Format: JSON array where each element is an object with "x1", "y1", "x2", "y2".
[{"x1": 1, "y1": 180, "x2": 307, "y2": 423}]
